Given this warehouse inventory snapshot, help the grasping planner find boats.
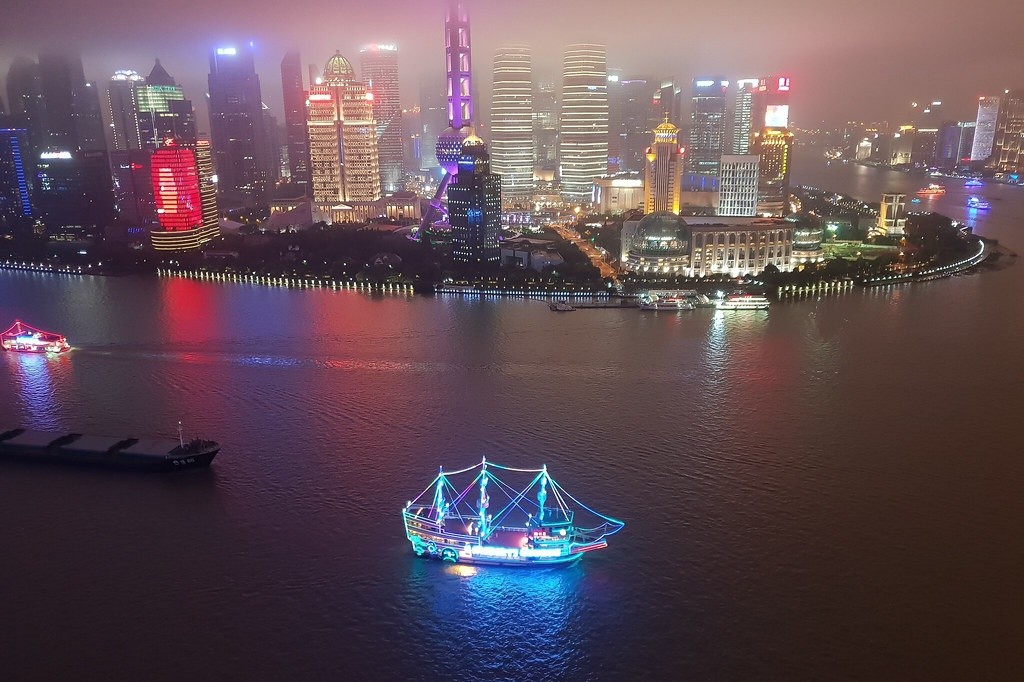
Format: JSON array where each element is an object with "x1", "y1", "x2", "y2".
[
  {"x1": 916, "y1": 188, "x2": 946, "y2": 200},
  {"x1": 965, "y1": 180, "x2": 983, "y2": 186},
  {"x1": 638, "y1": 296, "x2": 697, "y2": 310},
  {"x1": 714, "y1": 295, "x2": 770, "y2": 310},
  {"x1": 548, "y1": 300, "x2": 575, "y2": 311},
  {"x1": 0, "y1": 319, "x2": 71, "y2": 353},
  {"x1": 966, "y1": 196, "x2": 992, "y2": 210},
  {"x1": 928, "y1": 182, "x2": 945, "y2": 189},
  {"x1": 402, "y1": 456, "x2": 626, "y2": 566},
  {"x1": 911, "y1": 197, "x2": 921, "y2": 204}
]
